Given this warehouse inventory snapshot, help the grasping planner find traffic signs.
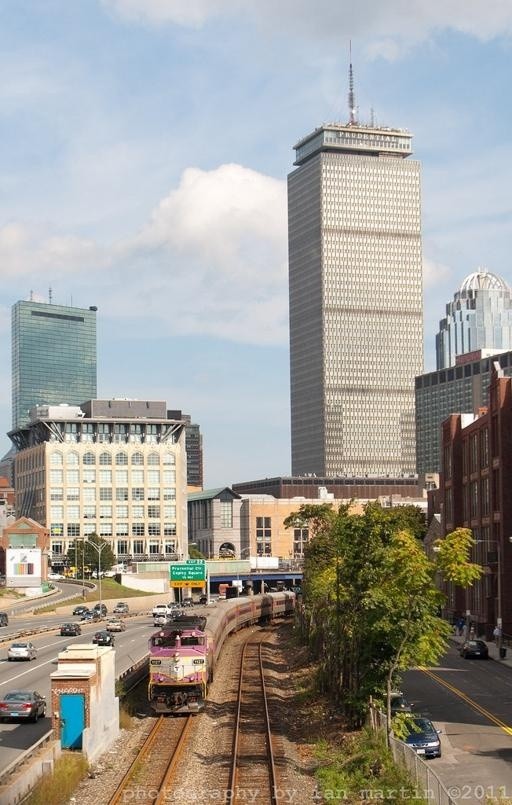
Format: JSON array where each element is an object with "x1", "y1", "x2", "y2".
[{"x1": 170, "y1": 565, "x2": 207, "y2": 581}]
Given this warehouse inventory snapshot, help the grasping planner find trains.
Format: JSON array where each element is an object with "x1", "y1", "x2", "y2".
[{"x1": 148, "y1": 590, "x2": 303, "y2": 714}]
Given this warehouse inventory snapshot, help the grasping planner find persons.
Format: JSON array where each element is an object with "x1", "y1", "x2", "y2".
[
  {"x1": 243, "y1": 583, "x2": 300, "y2": 598},
  {"x1": 493, "y1": 626, "x2": 502, "y2": 647},
  {"x1": 452, "y1": 616, "x2": 474, "y2": 640}
]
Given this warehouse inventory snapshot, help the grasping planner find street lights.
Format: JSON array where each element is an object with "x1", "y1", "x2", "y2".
[
  {"x1": 85, "y1": 539, "x2": 115, "y2": 621},
  {"x1": 221, "y1": 547, "x2": 252, "y2": 597}
]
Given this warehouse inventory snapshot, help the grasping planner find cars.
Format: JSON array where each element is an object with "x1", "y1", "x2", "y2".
[
  {"x1": 0, "y1": 611, "x2": 8, "y2": 627},
  {"x1": 460, "y1": 640, "x2": 488, "y2": 659},
  {"x1": 106, "y1": 618, "x2": 125, "y2": 632},
  {"x1": 390, "y1": 696, "x2": 442, "y2": 760},
  {"x1": 152, "y1": 594, "x2": 208, "y2": 627},
  {"x1": 93, "y1": 632, "x2": 116, "y2": 646},
  {"x1": 1, "y1": 690, "x2": 46, "y2": 722},
  {"x1": 8, "y1": 642, "x2": 38, "y2": 661},
  {"x1": 61, "y1": 623, "x2": 82, "y2": 636},
  {"x1": 113, "y1": 603, "x2": 129, "y2": 613},
  {"x1": 73, "y1": 603, "x2": 107, "y2": 621}
]
